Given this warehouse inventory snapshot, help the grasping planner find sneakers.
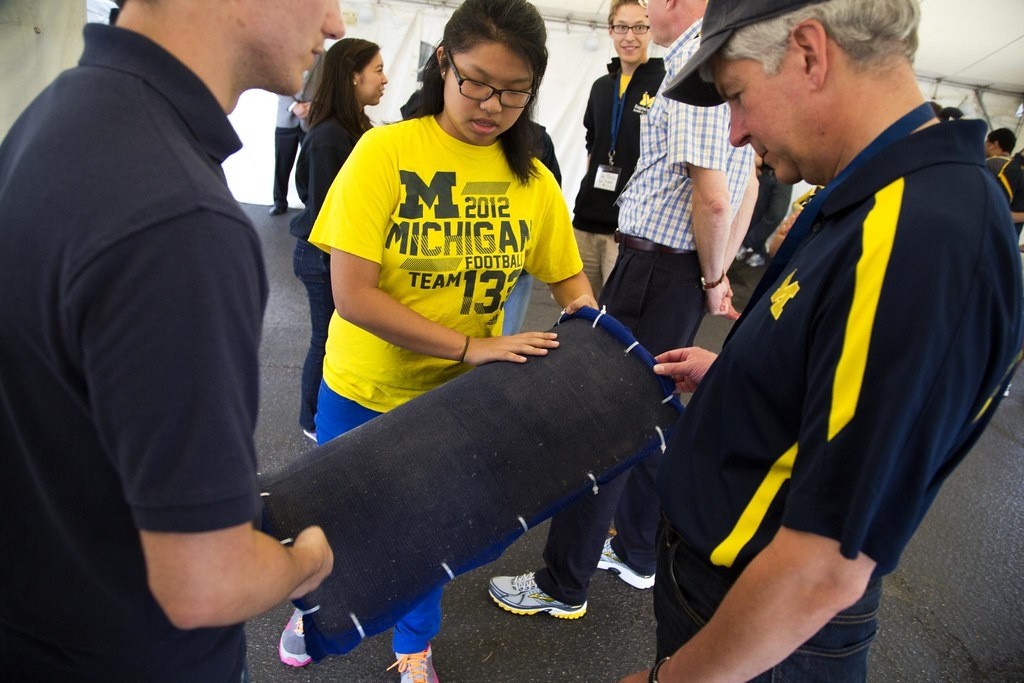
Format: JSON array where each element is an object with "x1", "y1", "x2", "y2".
[
  {"x1": 386, "y1": 641, "x2": 440, "y2": 683},
  {"x1": 597, "y1": 536, "x2": 656, "y2": 590},
  {"x1": 487, "y1": 571, "x2": 588, "y2": 620},
  {"x1": 278, "y1": 604, "x2": 321, "y2": 667}
]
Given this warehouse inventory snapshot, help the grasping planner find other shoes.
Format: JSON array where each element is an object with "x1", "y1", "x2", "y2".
[
  {"x1": 303, "y1": 428, "x2": 318, "y2": 442},
  {"x1": 269, "y1": 205, "x2": 288, "y2": 215},
  {"x1": 736, "y1": 243, "x2": 765, "y2": 268}
]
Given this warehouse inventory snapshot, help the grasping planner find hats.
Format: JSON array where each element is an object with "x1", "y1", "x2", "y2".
[{"x1": 662, "y1": 0, "x2": 815, "y2": 108}]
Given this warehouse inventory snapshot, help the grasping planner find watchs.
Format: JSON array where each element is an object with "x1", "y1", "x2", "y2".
[{"x1": 701, "y1": 269, "x2": 724, "y2": 290}]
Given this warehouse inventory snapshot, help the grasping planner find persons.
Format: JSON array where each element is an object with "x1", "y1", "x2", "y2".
[
  {"x1": 929, "y1": 101, "x2": 1024, "y2": 396},
  {"x1": 618, "y1": 0, "x2": 1024, "y2": 683},
  {"x1": 0, "y1": 0, "x2": 345, "y2": 683},
  {"x1": 268, "y1": 0, "x2": 666, "y2": 442},
  {"x1": 735, "y1": 157, "x2": 792, "y2": 267},
  {"x1": 489, "y1": 0, "x2": 762, "y2": 621},
  {"x1": 279, "y1": 0, "x2": 598, "y2": 683}
]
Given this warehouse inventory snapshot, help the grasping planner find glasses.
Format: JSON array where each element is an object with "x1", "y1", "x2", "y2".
[
  {"x1": 443, "y1": 44, "x2": 530, "y2": 108},
  {"x1": 610, "y1": 24, "x2": 650, "y2": 34}
]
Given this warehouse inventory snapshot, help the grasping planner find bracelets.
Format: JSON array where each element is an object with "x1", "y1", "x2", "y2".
[
  {"x1": 461, "y1": 336, "x2": 470, "y2": 362},
  {"x1": 649, "y1": 656, "x2": 670, "y2": 683}
]
